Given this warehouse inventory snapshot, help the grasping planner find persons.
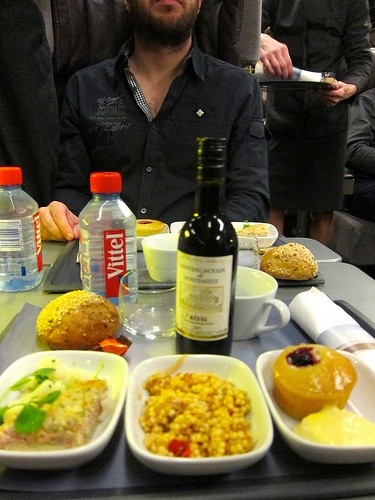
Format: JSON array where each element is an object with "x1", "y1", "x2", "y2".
[{"x1": 0, "y1": 0, "x2": 375, "y2": 241}]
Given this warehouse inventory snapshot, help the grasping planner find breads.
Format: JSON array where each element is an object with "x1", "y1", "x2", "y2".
[
  {"x1": 35, "y1": 289, "x2": 120, "y2": 351},
  {"x1": 260, "y1": 243, "x2": 319, "y2": 280}
]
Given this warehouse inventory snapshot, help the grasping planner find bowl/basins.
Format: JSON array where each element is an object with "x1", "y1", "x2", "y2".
[
  {"x1": 231, "y1": 221, "x2": 279, "y2": 249},
  {"x1": 256, "y1": 349, "x2": 375, "y2": 463},
  {"x1": 124, "y1": 354, "x2": 272, "y2": 475},
  {"x1": 137, "y1": 224, "x2": 170, "y2": 251},
  {"x1": 0, "y1": 350, "x2": 126, "y2": 471}
]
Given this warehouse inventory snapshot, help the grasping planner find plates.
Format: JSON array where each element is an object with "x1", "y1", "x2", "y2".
[{"x1": 258, "y1": 80, "x2": 335, "y2": 91}]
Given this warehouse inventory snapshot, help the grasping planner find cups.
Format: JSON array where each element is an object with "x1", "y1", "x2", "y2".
[
  {"x1": 141, "y1": 234, "x2": 181, "y2": 283},
  {"x1": 171, "y1": 222, "x2": 187, "y2": 233},
  {"x1": 232, "y1": 265, "x2": 291, "y2": 341},
  {"x1": 118, "y1": 268, "x2": 176, "y2": 344},
  {"x1": 238, "y1": 236, "x2": 259, "y2": 271}
]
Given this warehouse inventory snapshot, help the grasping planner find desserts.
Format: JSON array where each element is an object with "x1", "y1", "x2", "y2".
[
  {"x1": 136, "y1": 220, "x2": 166, "y2": 236},
  {"x1": 235, "y1": 223, "x2": 266, "y2": 236},
  {"x1": 273, "y1": 343, "x2": 358, "y2": 421}
]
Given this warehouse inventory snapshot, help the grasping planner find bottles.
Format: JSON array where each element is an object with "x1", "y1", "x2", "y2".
[
  {"x1": 0, "y1": 166, "x2": 44, "y2": 293},
  {"x1": 173, "y1": 136, "x2": 237, "y2": 357},
  {"x1": 79, "y1": 171, "x2": 139, "y2": 308}
]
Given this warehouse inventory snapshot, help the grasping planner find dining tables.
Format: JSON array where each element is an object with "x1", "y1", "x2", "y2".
[{"x1": 0, "y1": 237, "x2": 375, "y2": 500}]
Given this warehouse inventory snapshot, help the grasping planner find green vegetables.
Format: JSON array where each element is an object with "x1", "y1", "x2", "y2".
[{"x1": 0, "y1": 366, "x2": 61, "y2": 436}]
